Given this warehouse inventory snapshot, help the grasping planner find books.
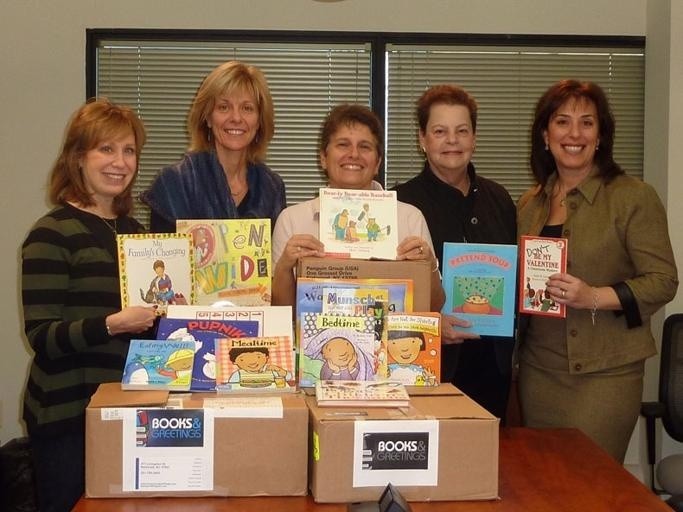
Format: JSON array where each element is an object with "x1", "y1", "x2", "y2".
[
  {"x1": 211, "y1": 335, "x2": 298, "y2": 395},
  {"x1": 295, "y1": 309, "x2": 376, "y2": 389},
  {"x1": 436, "y1": 238, "x2": 518, "y2": 339},
  {"x1": 114, "y1": 231, "x2": 201, "y2": 317},
  {"x1": 313, "y1": 185, "x2": 397, "y2": 262},
  {"x1": 292, "y1": 276, "x2": 414, "y2": 380},
  {"x1": 380, "y1": 310, "x2": 443, "y2": 387},
  {"x1": 118, "y1": 338, "x2": 195, "y2": 392},
  {"x1": 174, "y1": 214, "x2": 273, "y2": 312},
  {"x1": 516, "y1": 233, "x2": 568, "y2": 320},
  {"x1": 312, "y1": 375, "x2": 411, "y2": 408},
  {"x1": 156, "y1": 315, "x2": 258, "y2": 391}
]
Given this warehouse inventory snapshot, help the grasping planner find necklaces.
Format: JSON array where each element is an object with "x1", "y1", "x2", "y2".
[
  {"x1": 459, "y1": 174, "x2": 469, "y2": 195},
  {"x1": 229, "y1": 182, "x2": 246, "y2": 198},
  {"x1": 95, "y1": 207, "x2": 121, "y2": 244}
]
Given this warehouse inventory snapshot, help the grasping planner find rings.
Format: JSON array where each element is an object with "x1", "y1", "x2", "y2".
[
  {"x1": 297, "y1": 247, "x2": 300, "y2": 252},
  {"x1": 561, "y1": 290, "x2": 565, "y2": 298},
  {"x1": 418, "y1": 246, "x2": 423, "y2": 254}
]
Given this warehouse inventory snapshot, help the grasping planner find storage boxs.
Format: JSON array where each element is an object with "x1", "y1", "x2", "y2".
[
  {"x1": 305, "y1": 379, "x2": 501, "y2": 506},
  {"x1": 84, "y1": 381, "x2": 311, "y2": 499},
  {"x1": 295, "y1": 257, "x2": 433, "y2": 315}
]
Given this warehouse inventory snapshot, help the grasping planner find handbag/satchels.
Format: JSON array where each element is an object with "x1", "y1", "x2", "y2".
[{"x1": 507, "y1": 363, "x2": 526, "y2": 428}]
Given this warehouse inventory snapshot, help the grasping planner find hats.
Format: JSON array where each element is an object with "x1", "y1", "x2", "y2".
[{"x1": 164, "y1": 348, "x2": 195, "y2": 368}]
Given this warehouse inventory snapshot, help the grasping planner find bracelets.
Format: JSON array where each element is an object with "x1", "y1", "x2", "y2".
[
  {"x1": 105, "y1": 315, "x2": 112, "y2": 335},
  {"x1": 590, "y1": 285, "x2": 600, "y2": 321}
]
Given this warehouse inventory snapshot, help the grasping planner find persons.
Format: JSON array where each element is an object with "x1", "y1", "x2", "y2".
[
  {"x1": 261, "y1": 102, "x2": 446, "y2": 316},
  {"x1": 384, "y1": 81, "x2": 522, "y2": 426},
  {"x1": 135, "y1": 58, "x2": 288, "y2": 239},
  {"x1": 17, "y1": 98, "x2": 162, "y2": 511},
  {"x1": 508, "y1": 79, "x2": 680, "y2": 465}
]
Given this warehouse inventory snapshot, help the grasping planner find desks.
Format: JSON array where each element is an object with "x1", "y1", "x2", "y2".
[{"x1": 66, "y1": 424, "x2": 677, "y2": 512}]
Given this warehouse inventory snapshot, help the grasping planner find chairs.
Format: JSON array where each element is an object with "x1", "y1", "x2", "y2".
[{"x1": 639, "y1": 312, "x2": 683, "y2": 511}]
